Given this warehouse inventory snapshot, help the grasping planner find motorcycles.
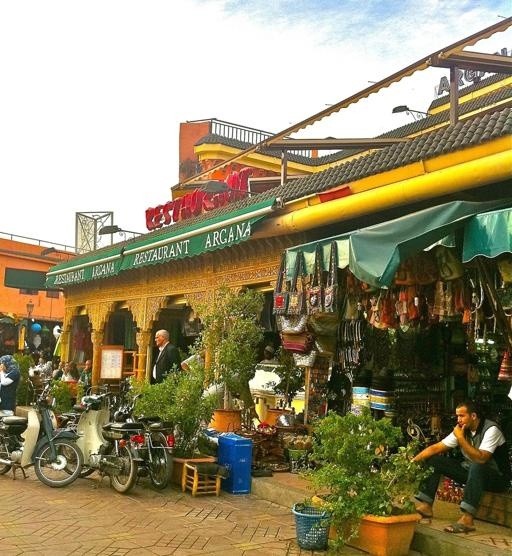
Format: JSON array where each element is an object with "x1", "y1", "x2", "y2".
[
  {"x1": 1, "y1": 370, "x2": 84, "y2": 488},
  {"x1": 58, "y1": 378, "x2": 174, "y2": 493}
]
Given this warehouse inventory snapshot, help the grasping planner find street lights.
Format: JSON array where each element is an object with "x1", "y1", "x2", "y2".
[
  {"x1": 99, "y1": 226, "x2": 145, "y2": 237},
  {"x1": 392, "y1": 105, "x2": 435, "y2": 116},
  {"x1": 199, "y1": 180, "x2": 269, "y2": 200}
]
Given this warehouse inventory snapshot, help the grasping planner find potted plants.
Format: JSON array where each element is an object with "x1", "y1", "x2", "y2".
[
  {"x1": 124, "y1": 364, "x2": 216, "y2": 487},
  {"x1": 48, "y1": 379, "x2": 73, "y2": 427},
  {"x1": 268, "y1": 345, "x2": 303, "y2": 426},
  {"x1": 195, "y1": 282, "x2": 267, "y2": 433},
  {"x1": 298, "y1": 406, "x2": 424, "y2": 556}
]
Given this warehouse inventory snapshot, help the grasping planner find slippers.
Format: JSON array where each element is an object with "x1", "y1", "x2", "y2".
[
  {"x1": 415, "y1": 509, "x2": 434, "y2": 518},
  {"x1": 444, "y1": 522, "x2": 476, "y2": 533}
]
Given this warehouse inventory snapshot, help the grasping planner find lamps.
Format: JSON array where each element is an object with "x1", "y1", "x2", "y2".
[
  {"x1": 99, "y1": 225, "x2": 143, "y2": 236},
  {"x1": 392, "y1": 106, "x2": 432, "y2": 116},
  {"x1": 199, "y1": 182, "x2": 259, "y2": 197}
]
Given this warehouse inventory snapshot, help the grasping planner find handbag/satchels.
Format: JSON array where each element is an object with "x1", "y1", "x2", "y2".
[
  {"x1": 313, "y1": 338, "x2": 338, "y2": 356},
  {"x1": 323, "y1": 284, "x2": 340, "y2": 313},
  {"x1": 293, "y1": 351, "x2": 318, "y2": 367},
  {"x1": 306, "y1": 288, "x2": 323, "y2": 316},
  {"x1": 272, "y1": 293, "x2": 287, "y2": 315},
  {"x1": 307, "y1": 314, "x2": 343, "y2": 336},
  {"x1": 274, "y1": 316, "x2": 307, "y2": 335},
  {"x1": 287, "y1": 291, "x2": 305, "y2": 316},
  {"x1": 280, "y1": 332, "x2": 317, "y2": 353}
]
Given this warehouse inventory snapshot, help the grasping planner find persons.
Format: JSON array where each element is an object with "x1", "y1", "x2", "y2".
[
  {"x1": 30, "y1": 354, "x2": 92, "y2": 408},
  {"x1": 0, "y1": 354, "x2": 21, "y2": 416},
  {"x1": 150, "y1": 329, "x2": 181, "y2": 384},
  {"x1": 409, "y1": 398, "x2": 511, "y2": 533},
  {"x1": 181, "y1": 350, "x2": 206, "y2": 372},
  {"x1": 260, "y1": 343, "x2": 280, "y2": 363}
]
